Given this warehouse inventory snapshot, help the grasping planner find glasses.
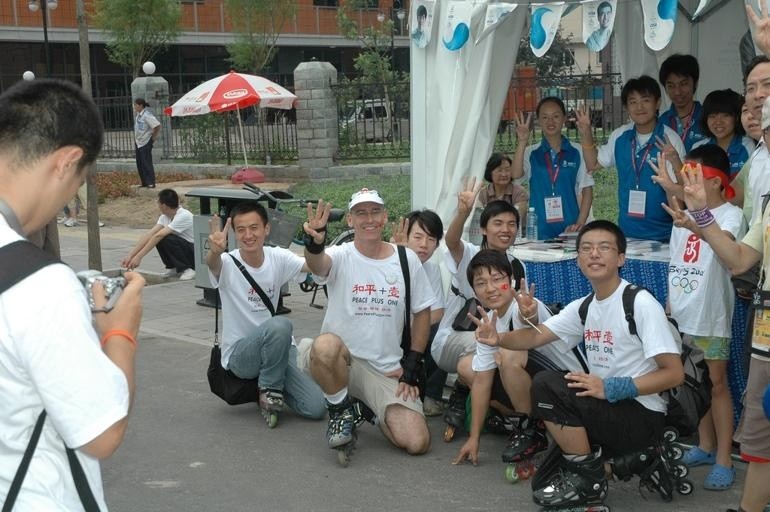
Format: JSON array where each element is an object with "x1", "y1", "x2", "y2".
[
  {"x1": 472, "y1": 274, "x2": 507, "y2": 288},
  {"x1": 577, "y1": 245, "x2": 619, "y2": 256}
]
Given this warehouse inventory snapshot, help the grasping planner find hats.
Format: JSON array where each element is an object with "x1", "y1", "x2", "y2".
[{"x1": 348, "y1": 189, "x2": 384, "y2": 211}]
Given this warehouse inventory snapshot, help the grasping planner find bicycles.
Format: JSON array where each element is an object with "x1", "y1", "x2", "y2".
[{"x1": 241, "y1": 180, "x2": 355, "y2": 310}]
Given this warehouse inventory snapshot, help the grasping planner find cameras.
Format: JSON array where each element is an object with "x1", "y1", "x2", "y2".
[{"x1": 78, "y1": 270, "x2": 126, "y2": 312}]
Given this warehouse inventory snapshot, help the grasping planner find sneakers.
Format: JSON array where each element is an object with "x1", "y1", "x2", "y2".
[
  {"x1": 179, "y1": 268, "x2": 195, "y2": 280},
  {"x1": 161, "y1": 268, "x2": 177, "y2": 278},
  {"x1": 422, "y1": 396, "x2": 442, "y2": 415},
  {"x1": 57, "y1": 217, "x2": 104, "y2": 227}
]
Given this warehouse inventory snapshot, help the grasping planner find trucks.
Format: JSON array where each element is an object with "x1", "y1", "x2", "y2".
[
  {"x1": 496, "y1": 61, "x2": 541, "y2": 134},
  {"x1": 545, "y1": 77, "x2": 604, "y2": 129}
]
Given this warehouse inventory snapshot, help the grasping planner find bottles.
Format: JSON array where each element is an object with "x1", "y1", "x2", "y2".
[
  {"x1": 559, "y1": 247, "x2": 571, "y2": 261},
  {"x1": 526, "y1": 207, "x2": 538, "y2": 240}
]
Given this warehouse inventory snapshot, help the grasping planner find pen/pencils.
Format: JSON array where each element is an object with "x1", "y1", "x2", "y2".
[{"x1": 544, "y1": 240, "x2": 567, "y2": 243}]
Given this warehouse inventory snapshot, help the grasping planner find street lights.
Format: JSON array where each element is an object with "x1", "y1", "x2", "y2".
[
  {"x1": 27, "y1": 0, "x2": 58, "y2": 78},
  {"x1": 375, "y1": 3, "x2": 407, "y2": 81}
]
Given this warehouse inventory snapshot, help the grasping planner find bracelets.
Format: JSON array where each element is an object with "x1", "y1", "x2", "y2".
[
  {"x1": 101, "y1": 330, "x2": 139, "y2": 350},
  {"x1": 603, "y1": 376, "x2": 642, "y2": 403},
  {"x1": 687, "y1": 204, "x2": 719, "y2": 229}
]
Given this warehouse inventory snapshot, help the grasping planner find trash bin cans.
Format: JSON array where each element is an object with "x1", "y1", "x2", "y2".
[{"x1": 184, "y1": 188, "x2": 294, "y2": 316}]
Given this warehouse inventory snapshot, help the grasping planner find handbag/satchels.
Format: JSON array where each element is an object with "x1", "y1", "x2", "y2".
[
  {"x1": 731, "y1": 265, "x2": 760, "y2": 301},
  {"x1": 207, "y1": 345, "x2": 257, "y2": 405}
]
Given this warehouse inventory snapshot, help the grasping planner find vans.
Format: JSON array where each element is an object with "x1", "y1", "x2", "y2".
[{"x1": 335, "y1": 98, "x2": 397, "y2": 146}]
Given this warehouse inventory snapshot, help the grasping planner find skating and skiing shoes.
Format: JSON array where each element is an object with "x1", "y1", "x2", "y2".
[
  {"x1": 325, "y1": 393, "x2": 358, "y2": 468},
  {"x1": 534, "y1": 444, "x2": 610, "y2": 512},
  {"x1": 443, "y1": 381, "x2": 470, "y2": 442},
  {"x1": 351, "y1": 397, "x2": 375, "y2": 426},
  {"x1": 610, "y1": 427, "x2": 691, "y2": 503},
  {"x1": 502, "y1": 412, "x2": 548, "y2": 482},
  {"x1": 260, "y1": 388, "x2": 285, "y2": 427}
]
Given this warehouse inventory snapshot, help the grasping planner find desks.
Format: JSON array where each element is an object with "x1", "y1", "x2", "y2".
[{"x1": 505, "y1": 237, "x2": 670, "y2": 308}]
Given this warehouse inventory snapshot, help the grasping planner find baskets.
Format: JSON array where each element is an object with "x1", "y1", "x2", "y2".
[{"x1": 264, "y1": 207, "x2": 301, "y2": 250}]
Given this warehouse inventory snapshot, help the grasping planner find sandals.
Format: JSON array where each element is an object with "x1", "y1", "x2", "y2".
[
  {"x1": 680, "y1": 446, "x2": 716, "y2": 467},
  {"x1": 703, "y1": 463, "x2": 736, "y2": 490}
]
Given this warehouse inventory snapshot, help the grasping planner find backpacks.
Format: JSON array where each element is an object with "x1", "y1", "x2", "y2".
[{"x1": 578, "y1": 284, "x2": 713, "y2": 437}]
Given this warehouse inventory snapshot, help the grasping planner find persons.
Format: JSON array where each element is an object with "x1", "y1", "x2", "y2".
[
  {"x1": 468, "y1": 219, "x2": 692, "y2": 511},
  {"x1": 386, "y1": 176, "x2": 589, "y2": 485},
  {"x1": 410, "y1": 4, "x2": 429, "y2": 47},
  {"x1": 647, "y1": 0, "x2": 770, "y2": 490},
  {"x1": 678, "y1": 94, "x2": 770, "y2": 512},
  {"x1": 301, "y1": 189, "x2": 437, "y2": 465},
  {"x1": 584, "y1": 2, "x2": 612, "y2": 52},
  {"x1": 119, "y1": 189, "x2": 196, "y2": 281},
  {"x1": 0, "y1": 80, "x2": 148, "y2": 511},
  {"x1": 479, "y1": 52, "x2": 758, "y2": 245},
  {"x1": 59, "y1": 182, "x2": 105, "y2": 227},
  {"x1": 201, "y1": 204, "x2": 326, "y2": 429},
  {"x1": 132, "y1": 98, "x2": 161, "y2": 188}
]
